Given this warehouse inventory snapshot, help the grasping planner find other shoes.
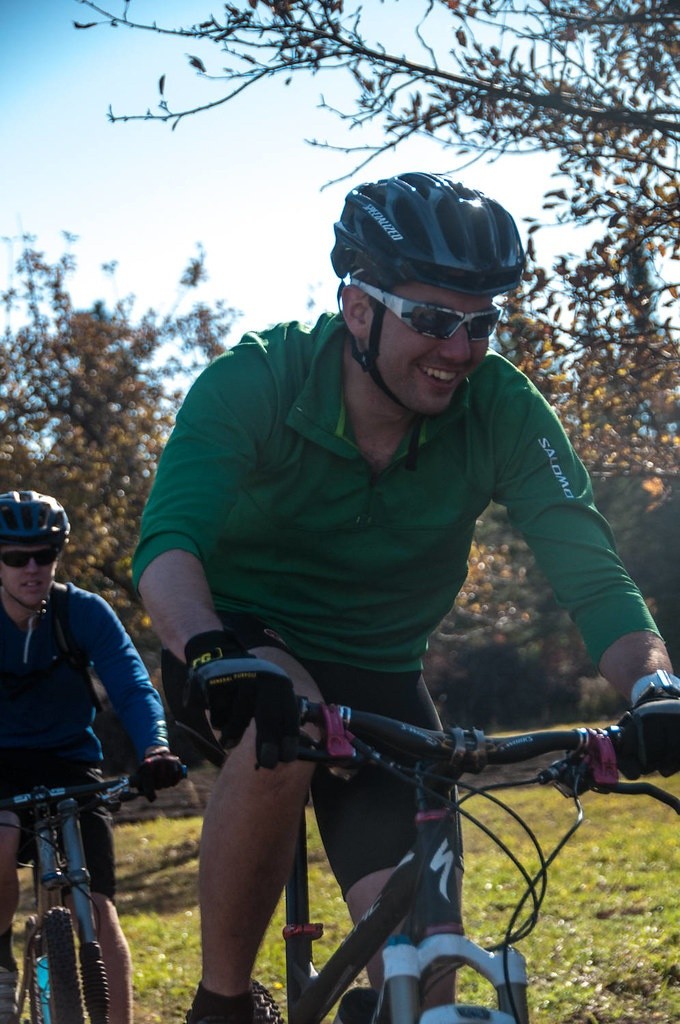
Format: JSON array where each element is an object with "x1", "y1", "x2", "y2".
[{"x1": 0, "y1": 959, "x2": 19, "y2": 1024}]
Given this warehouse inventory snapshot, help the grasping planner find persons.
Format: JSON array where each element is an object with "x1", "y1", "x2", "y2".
[
  {"x1": 0, "y1": 490, "x2": 181, "y2": 1024},
  {"x1": 133, "y1": 173, "x2": 680, "y2": 1024}
]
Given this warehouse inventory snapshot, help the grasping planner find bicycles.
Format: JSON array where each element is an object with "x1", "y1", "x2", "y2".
[
  {"x1": 0, "y1": 764, "x2": 189, "y2": 1024},
  {"x1": 282, "y1": 696, "x2": 680, "y2": 1022}
]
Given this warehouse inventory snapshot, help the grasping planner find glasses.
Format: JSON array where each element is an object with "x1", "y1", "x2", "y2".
[
  {"x1": 0, "y1": 548, "x2": 59, "y2": 568},
  {"x1": 350, "y1": 277, "x2": 501, "y2": 341}
]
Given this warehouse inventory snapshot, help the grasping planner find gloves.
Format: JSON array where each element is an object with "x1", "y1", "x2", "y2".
[
  {"x1": 129, "y1": 751, "x2": 186, "y2": 802},
  {"x1": 184, "y1": 630, "x2": 302, "y2": 769},
  {"x1": 609, "y1": 669, "x2": 680, "y2": 781}
]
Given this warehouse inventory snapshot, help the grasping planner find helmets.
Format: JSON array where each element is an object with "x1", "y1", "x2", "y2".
[
  {"x1": 331, "y1": 171, "x2": 526, "y2": 297},
  {"x1": 0, "y1": 490, "x2": 71, "y2": 546}
]
